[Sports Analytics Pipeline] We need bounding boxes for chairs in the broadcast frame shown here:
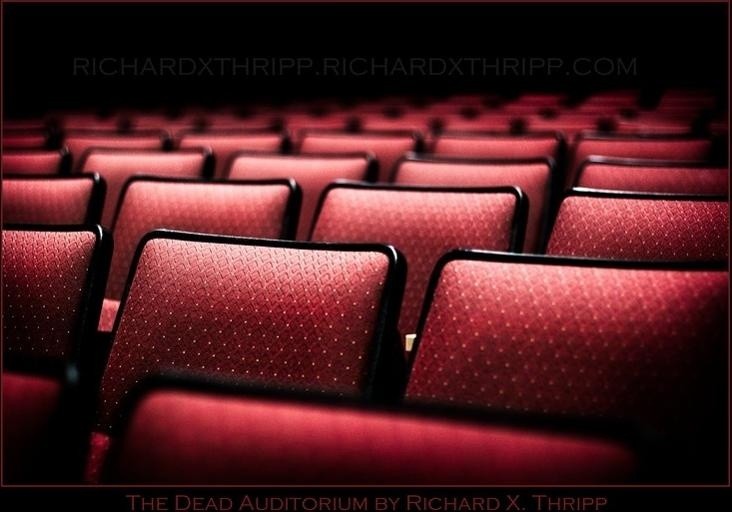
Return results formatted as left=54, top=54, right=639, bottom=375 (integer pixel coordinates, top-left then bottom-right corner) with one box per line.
left=0, top=92, right=732, bottom=486
